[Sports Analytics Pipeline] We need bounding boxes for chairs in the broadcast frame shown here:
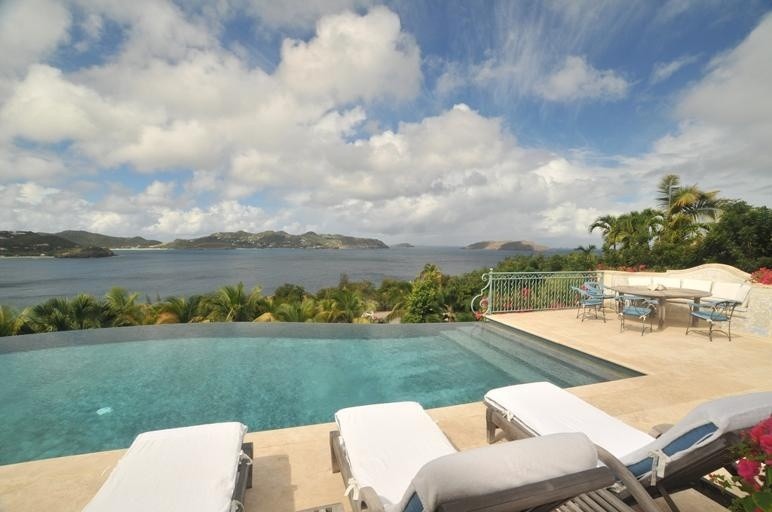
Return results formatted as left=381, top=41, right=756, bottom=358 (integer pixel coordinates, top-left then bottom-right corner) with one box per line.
left=569, top=280, right=741, bottom=345
left=483, top=380, right=772, bottom=511
left=329, top=401, right=664, bottom=512
left=81, top=422, right=253, bottom=512
